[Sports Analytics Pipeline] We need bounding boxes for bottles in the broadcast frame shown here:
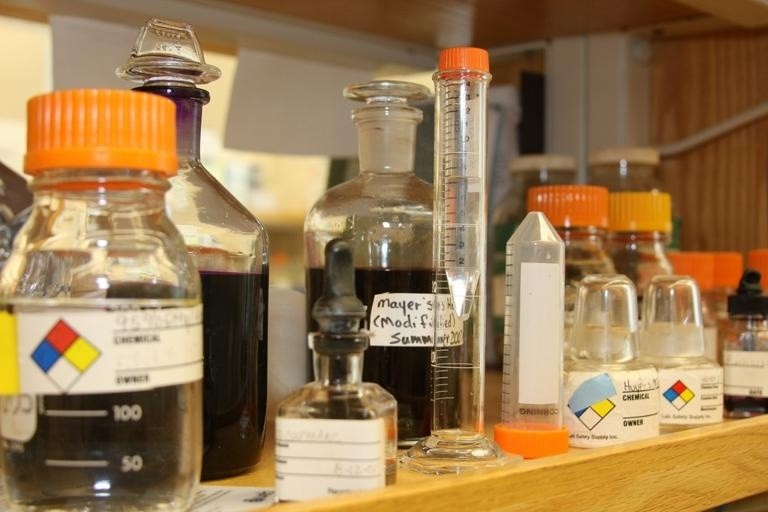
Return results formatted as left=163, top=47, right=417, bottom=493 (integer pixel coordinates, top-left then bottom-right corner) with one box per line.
left=113, top=20, right=273, bottom=481
left=0, top=88, right=204, bottom=512
left=300, top=79, right=474, bottom=450
left=638, top=276, right=722, bottom=436
left=275, top=238, right=400, bottom=499
left=721, top=270, right=767, bottom=420
left=523, top=185, right=619, bottom=351
left=606, top=188, right=679, bottom=321
left=562, top=276, right=660, bottom=453
